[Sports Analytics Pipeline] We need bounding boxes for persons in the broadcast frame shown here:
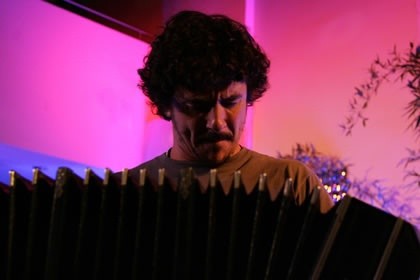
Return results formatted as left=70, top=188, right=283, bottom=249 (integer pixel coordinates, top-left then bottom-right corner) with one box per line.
left=111, top=10, right=335, bottom=212
